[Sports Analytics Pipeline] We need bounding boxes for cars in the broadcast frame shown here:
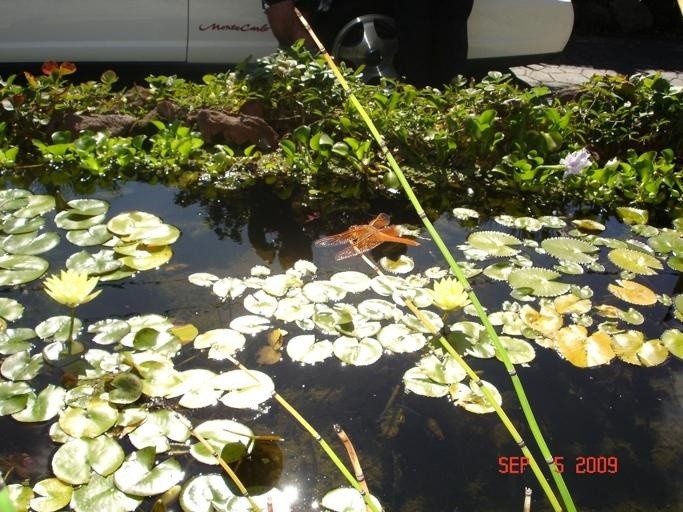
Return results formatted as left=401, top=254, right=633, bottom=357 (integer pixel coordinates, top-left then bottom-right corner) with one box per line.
left=0, top=1, right=575, bottom=87
